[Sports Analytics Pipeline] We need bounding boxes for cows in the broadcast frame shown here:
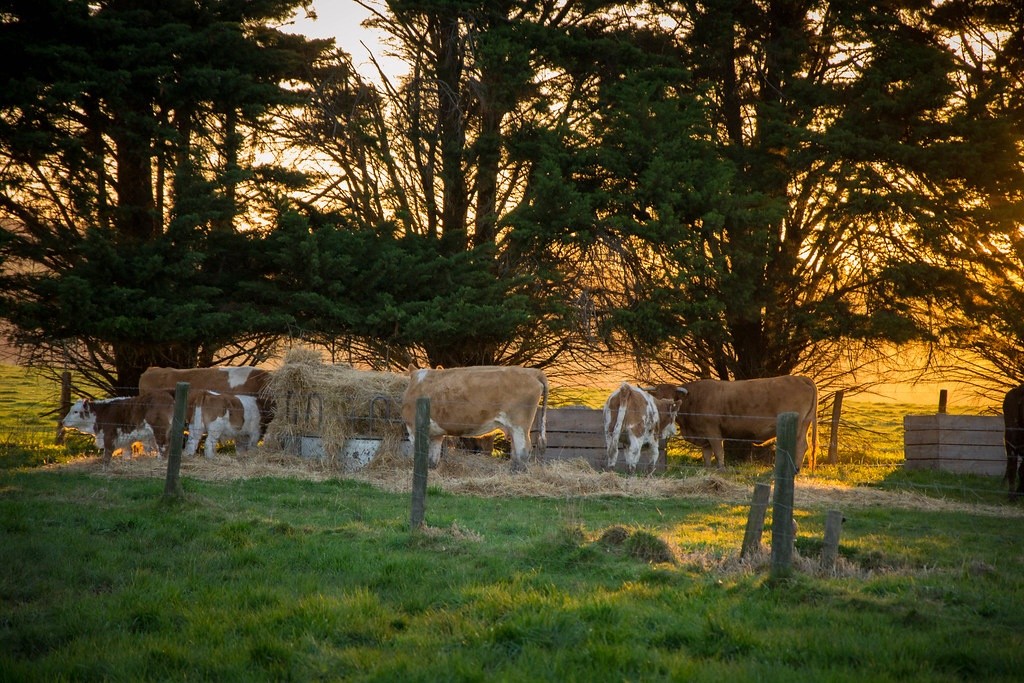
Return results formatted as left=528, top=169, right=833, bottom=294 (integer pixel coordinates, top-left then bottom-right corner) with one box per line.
left=62, top=367, right=277, bottom=471
left=603, top=382, right=683, bottom=476
left=637, top=376, right=818, bottom=476
left=403, top=364, right=549, bottom=473
